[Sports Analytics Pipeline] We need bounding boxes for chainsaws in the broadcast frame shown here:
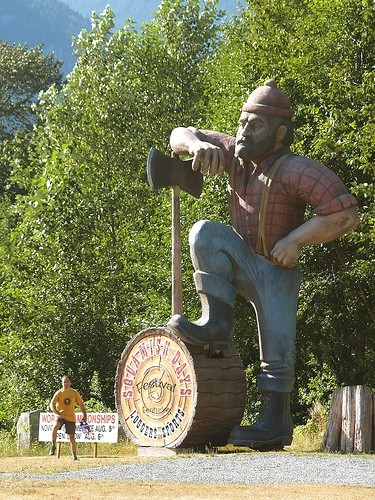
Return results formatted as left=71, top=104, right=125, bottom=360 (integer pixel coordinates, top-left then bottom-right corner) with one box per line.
left=79, top=419, right=90, bottom=434
